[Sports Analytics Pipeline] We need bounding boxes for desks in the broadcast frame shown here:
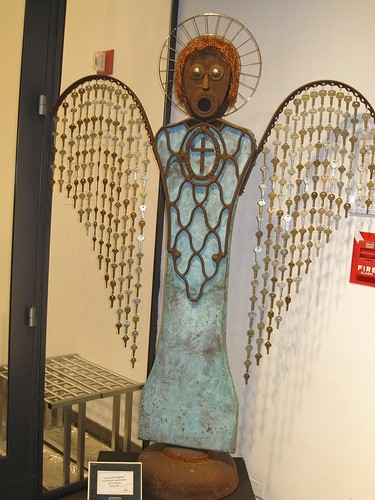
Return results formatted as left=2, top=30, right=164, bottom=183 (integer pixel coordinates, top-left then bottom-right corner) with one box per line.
left=93, top=450, right=256, bottom=500
